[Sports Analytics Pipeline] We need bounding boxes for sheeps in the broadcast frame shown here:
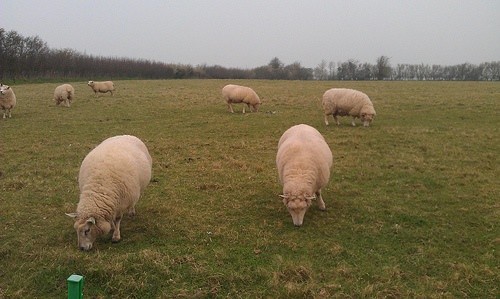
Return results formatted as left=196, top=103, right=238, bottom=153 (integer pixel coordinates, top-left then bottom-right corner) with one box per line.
left=89, top=80, right=116, bottom=97
left=66, top=134, right=153, bottom=251
left=275, top=124, right=333, bottom=227
left=0, top=82, right=16, bottom=119
left=322, top=89, right=375, bottom=127
left=54, top=84, right=75, bottom=107
left=222, top=84, right=262, bottom=114
